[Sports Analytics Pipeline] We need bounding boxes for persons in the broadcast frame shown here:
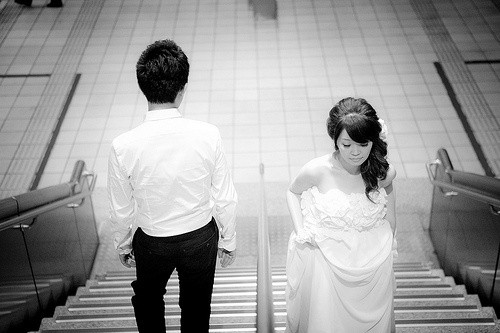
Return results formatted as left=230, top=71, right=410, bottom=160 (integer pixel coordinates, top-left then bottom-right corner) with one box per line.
left=106, top=37, right=237, bottom=333
left=285, top=96, right=398, bottom=333
